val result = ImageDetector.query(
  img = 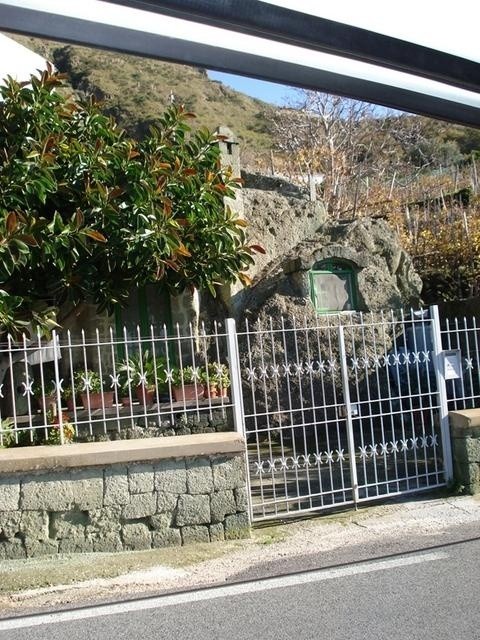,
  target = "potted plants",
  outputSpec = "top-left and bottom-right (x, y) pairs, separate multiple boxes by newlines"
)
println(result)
(74, 369), (103, 409)
(62, 387), (76, 411)
(206, 363), (231, 397)
(119, 386), (131, 405)
(99, 381), (115, 408)
(116, 350), (160, 407)
(201, 368), (218, 398)
(169, 364), (204, 402)
(33, 375), (59, 416)
